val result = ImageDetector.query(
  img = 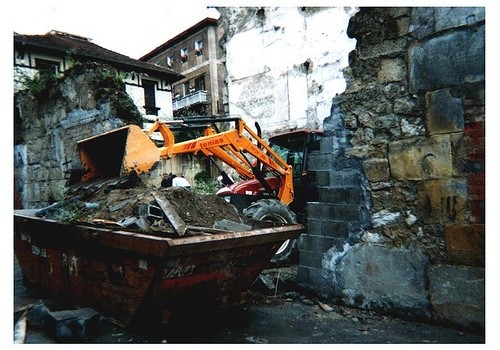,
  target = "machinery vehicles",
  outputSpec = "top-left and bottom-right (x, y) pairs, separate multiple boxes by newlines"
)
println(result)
(69, 113), (325, 266)
